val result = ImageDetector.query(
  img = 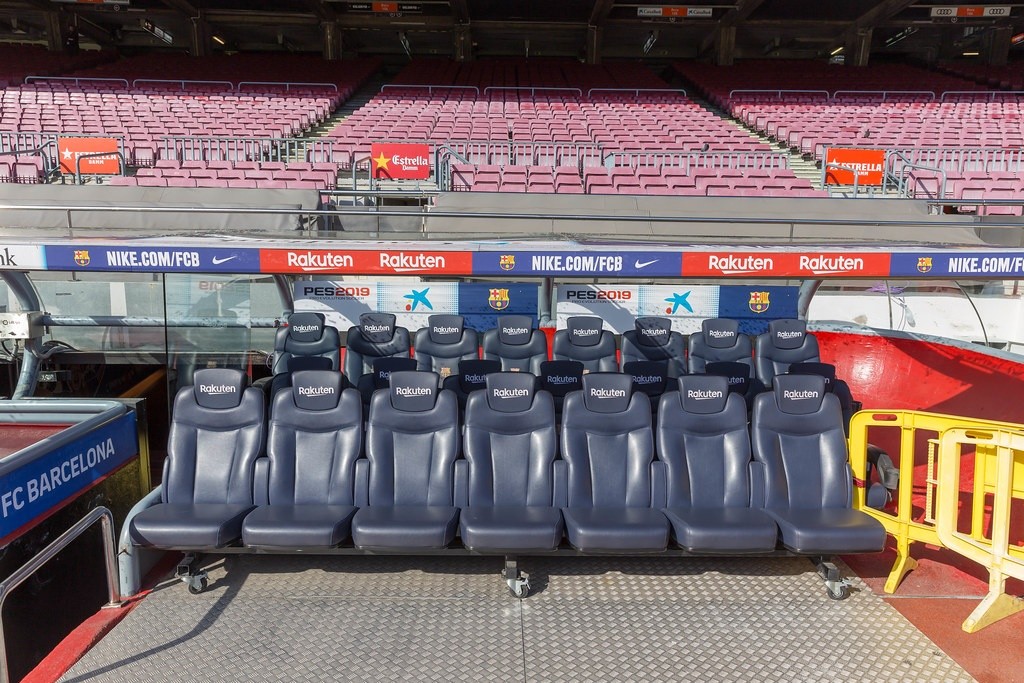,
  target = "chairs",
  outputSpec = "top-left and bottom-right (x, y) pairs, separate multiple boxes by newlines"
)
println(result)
(119, 313), (900, 602)
(0, 66), (1024, 217)
